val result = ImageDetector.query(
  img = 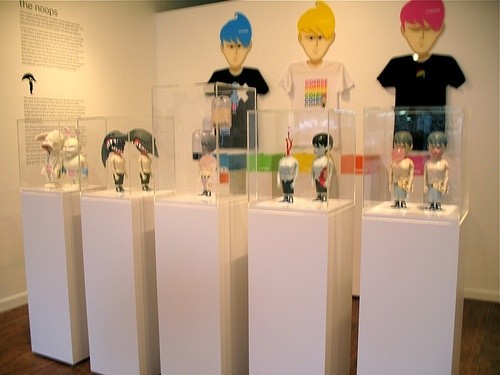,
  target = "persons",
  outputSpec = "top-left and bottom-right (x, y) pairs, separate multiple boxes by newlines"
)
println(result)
(110, 151), (128, 191)
(277, 154), (299, 203)
(139, 152), (153, 190)
(423, 131), (450, 209)
(313, 133), (333, 201)
(198, 136), (217, 196)
(389, 131), (415, 208)
